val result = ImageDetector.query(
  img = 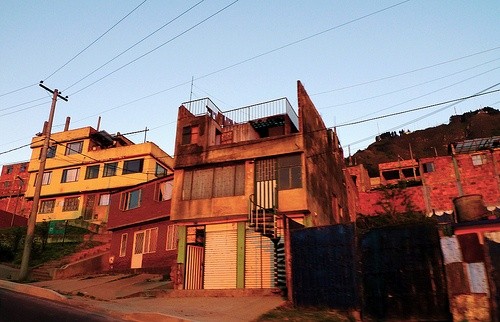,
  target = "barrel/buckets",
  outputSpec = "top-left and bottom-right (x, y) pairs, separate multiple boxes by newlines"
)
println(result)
(453, 194), (483, 222)
